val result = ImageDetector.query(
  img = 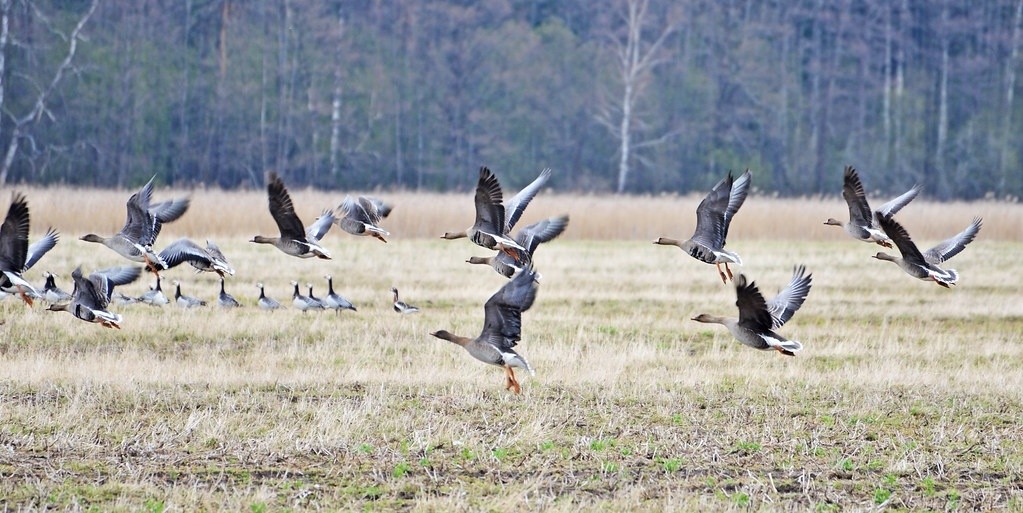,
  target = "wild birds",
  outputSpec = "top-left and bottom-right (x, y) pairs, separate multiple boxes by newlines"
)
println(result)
(690, 263), (813, 357)
(440, 165), (571, 282)
(428, 261), (539, 395)
(822, 166), (923, 248)
(871, 209), (985, 289)
(652, 166), (754, 285)
(1, 169), (422, 331)
